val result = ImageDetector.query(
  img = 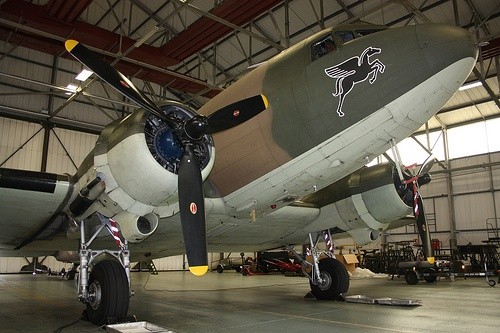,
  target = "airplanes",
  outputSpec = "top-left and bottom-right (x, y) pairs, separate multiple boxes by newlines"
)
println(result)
(6, 22), (481, 323)
